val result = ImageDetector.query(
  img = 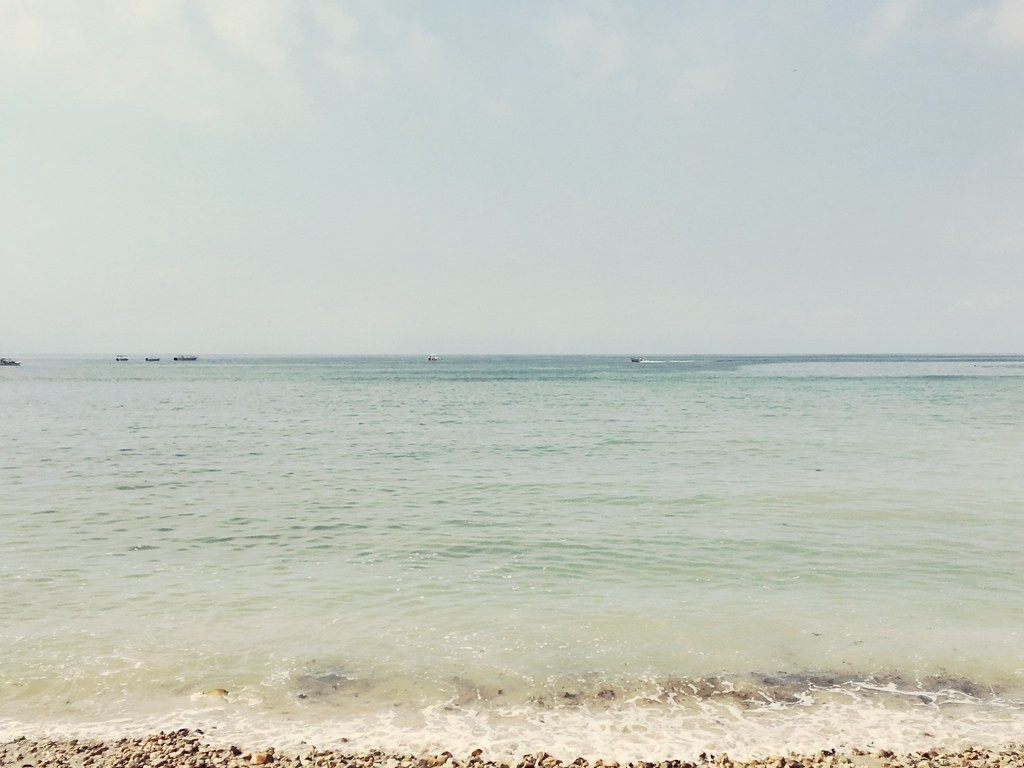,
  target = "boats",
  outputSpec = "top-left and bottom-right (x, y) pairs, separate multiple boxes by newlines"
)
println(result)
(630, 357), (643, 362)
(115, 355), (129, 362)
(145, 357), (161, 362)
(174, 355), (198, 361)
(0, 358), (22, 366)
(428, 354), (439, 361)
